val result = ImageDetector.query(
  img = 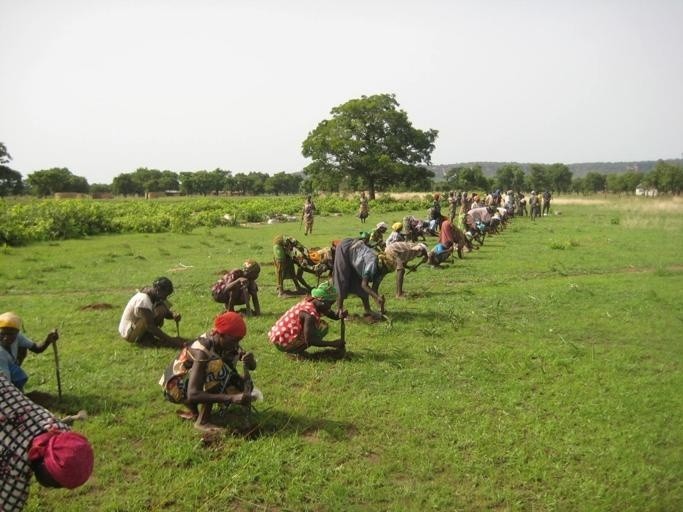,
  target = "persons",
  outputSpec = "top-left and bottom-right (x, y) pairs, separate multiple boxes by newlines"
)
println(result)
(332, 237), (397, 315)
(308, 239), (341, 278)
(273, 234), (323, 297)
(359, 222), (386, 253)
(385, 188), (551, 268)
(358, 192), (369, 225)
(212, 260), (261, 312)
(118, 276), (181, 343)
(158, 311), (256, 433)
(301, 196), (316, 235)
(386, 240), (429, 300)
(268, 281), (349, 356)
(0, 375), (95, 512)
(0, 310), (60, 390)
